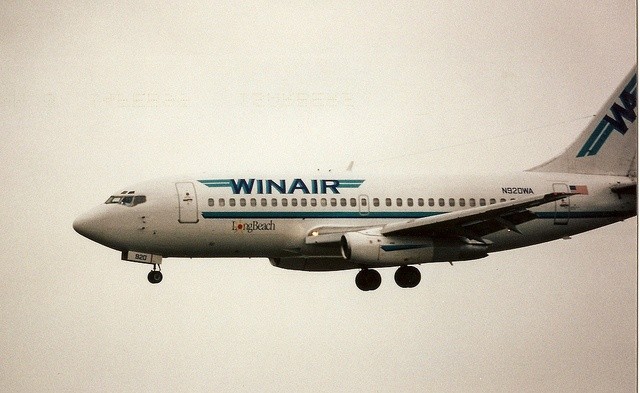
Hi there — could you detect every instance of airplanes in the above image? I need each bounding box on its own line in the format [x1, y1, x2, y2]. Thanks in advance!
[72, 62, 638, 291]
